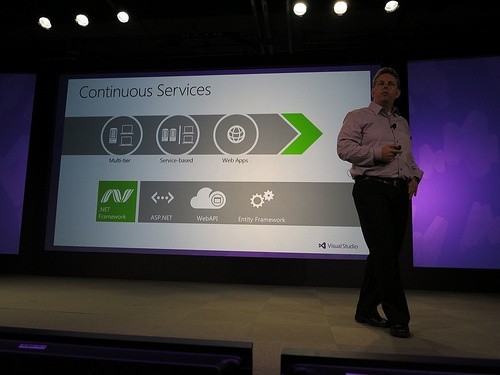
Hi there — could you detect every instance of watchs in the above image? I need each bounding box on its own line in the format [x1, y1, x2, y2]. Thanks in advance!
[412, 176, 420, 184]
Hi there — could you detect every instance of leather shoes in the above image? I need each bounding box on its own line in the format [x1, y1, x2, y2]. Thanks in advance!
[390, 320, 409, 338]
[356, 311, 391, 328]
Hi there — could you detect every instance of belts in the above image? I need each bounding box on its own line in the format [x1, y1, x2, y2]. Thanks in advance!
[356, 174, 407, 188]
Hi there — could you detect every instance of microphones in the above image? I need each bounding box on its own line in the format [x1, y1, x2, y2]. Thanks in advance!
[390, 123, 396, 128]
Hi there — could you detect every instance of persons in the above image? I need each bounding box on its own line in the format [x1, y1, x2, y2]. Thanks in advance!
[337, 68, 424, 339]
